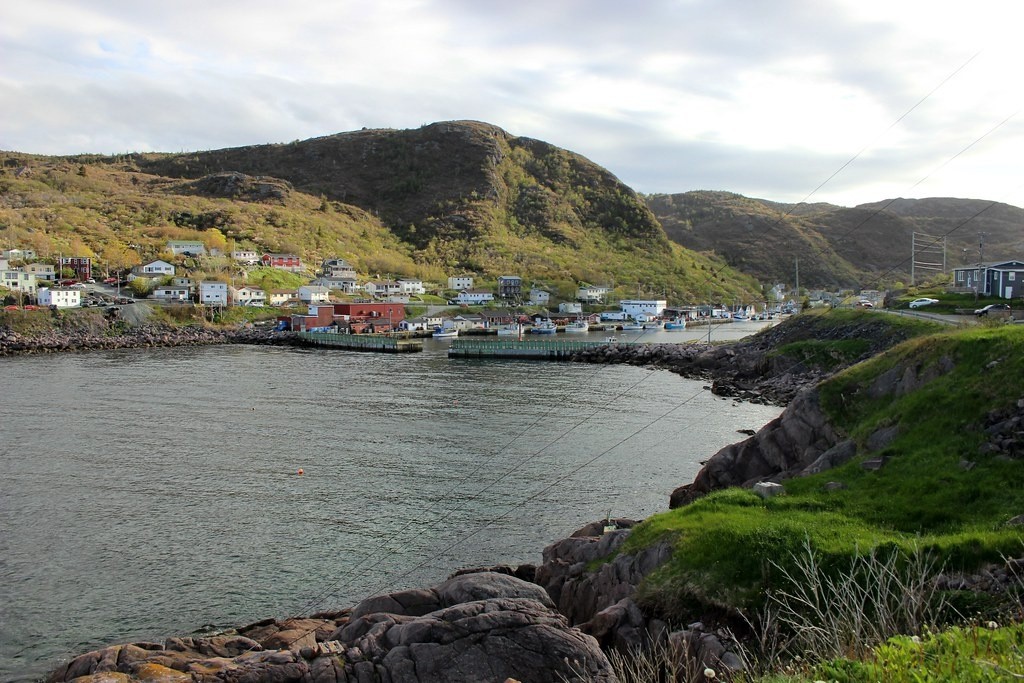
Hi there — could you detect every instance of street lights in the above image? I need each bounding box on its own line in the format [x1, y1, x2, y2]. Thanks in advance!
[389, 308, 393, 337]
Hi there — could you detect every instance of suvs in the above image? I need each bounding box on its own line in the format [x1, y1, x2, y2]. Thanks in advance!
[975, 304, 1010, 316]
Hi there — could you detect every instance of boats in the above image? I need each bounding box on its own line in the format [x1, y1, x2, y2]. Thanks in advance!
[643, 321, 664, 328]
[530, 320, 557, 334]
[432, 327, 459, 336]
[664, 315, 686, 329]
[565, 316, 589, 332]
[497, 322, 524, 336]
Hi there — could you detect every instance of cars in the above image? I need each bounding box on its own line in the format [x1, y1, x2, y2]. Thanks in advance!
[909, 298, 938, 308]
[857, 300, 873, 308]
